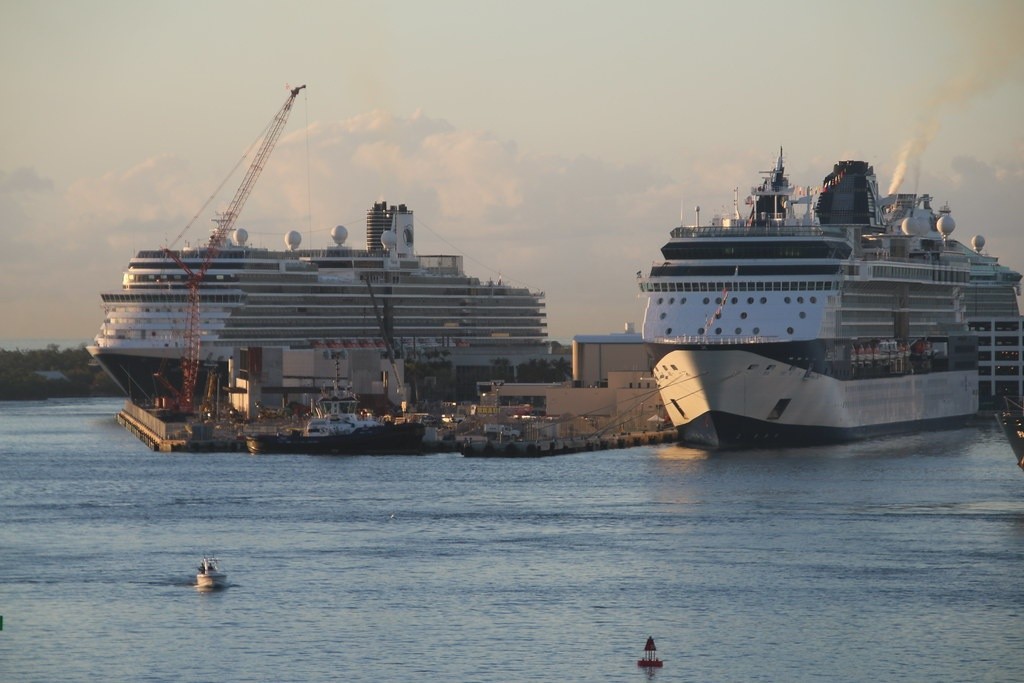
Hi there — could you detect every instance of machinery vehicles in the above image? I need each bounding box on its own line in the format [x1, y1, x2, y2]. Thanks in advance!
[197, 368, 218, 422]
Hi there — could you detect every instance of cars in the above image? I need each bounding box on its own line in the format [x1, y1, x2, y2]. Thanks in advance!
[407, 413, 439, 427]
[442, 414, 466, 424]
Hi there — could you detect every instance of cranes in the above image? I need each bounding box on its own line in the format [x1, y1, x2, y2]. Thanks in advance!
[151, 84, 309, 422]
[359, 274, 418, 424]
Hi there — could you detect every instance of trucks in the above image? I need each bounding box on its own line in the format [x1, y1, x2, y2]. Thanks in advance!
[483, 424, 520, 441]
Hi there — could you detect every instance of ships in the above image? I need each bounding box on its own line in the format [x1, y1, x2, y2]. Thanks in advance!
[202, 203, 574, 407]
[641, 146, 1024, 448]
[85, 225, 466, 400]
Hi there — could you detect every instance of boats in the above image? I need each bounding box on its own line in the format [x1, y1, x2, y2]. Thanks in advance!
[871, 342, 881, 368]
[990, 393, 1024, 473]
[244, 381, 427, 455]
[912, 337, 937, 361]
[849, 343, 857, 372]
[857, 342, 865, 372]
[878, 340, 912, 367]
[195, 553, 227, 592]
[864, 341, 873, 368]
